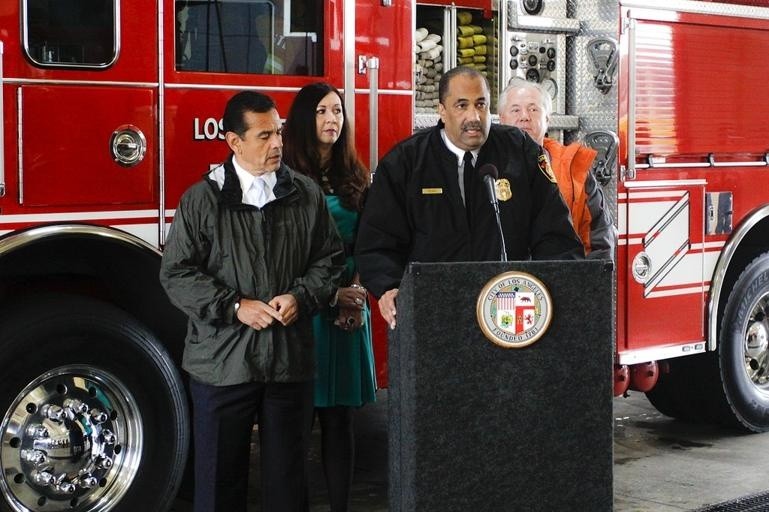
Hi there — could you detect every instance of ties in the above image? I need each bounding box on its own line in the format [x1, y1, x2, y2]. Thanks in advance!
[463, 152, 475, 222]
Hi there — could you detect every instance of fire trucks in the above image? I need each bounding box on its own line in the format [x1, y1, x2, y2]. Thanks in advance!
[1, 0, 768, 511]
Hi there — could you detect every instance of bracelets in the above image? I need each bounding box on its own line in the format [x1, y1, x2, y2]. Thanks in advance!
[350, 282, 365, 291]
[328, 288, 339, 309]
[234, 293, 244, 316]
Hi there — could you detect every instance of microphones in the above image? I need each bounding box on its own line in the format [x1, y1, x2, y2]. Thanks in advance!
[478, 161, 501, 218]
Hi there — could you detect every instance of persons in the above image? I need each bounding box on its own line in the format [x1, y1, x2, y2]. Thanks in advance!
[350, 66, 588, 332]
[158, 86, 349, 511]
[279, 82, 381, 512]
[498, 78, 616, 264]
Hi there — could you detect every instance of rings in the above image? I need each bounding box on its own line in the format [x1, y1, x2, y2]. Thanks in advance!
[346, 318, 356, 327]
[355, 297, 364, 305]
